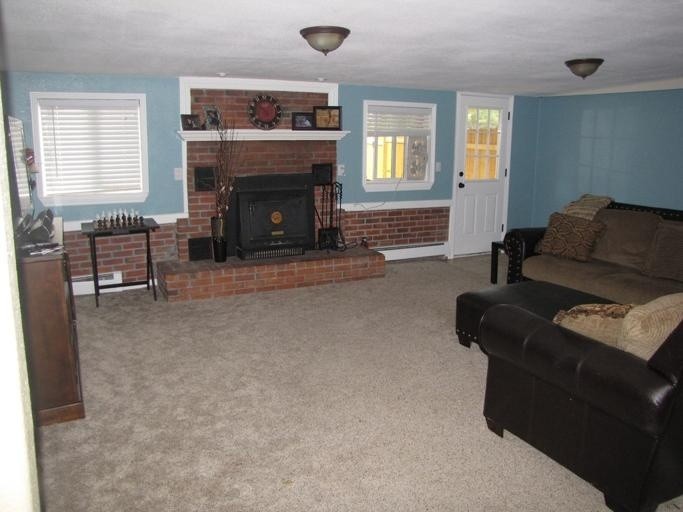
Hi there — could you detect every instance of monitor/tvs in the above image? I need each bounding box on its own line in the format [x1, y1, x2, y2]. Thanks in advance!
[4, 112, 33, 218]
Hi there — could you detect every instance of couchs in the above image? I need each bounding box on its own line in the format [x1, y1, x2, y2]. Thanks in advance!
[504, 194, 683, 306]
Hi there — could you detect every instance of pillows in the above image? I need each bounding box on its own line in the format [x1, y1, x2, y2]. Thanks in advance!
[534, 212, 605, 262]
[552, 304, 632, 348]
[621, 292, 683, 361]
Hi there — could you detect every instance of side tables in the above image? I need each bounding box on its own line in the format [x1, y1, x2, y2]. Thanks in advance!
[81, 218, 159, 307]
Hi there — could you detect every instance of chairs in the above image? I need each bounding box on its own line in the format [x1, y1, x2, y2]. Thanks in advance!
[477, 304, 683, 512]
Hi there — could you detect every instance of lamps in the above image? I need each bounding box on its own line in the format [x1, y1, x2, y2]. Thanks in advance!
[564, 58, 604, 80]
[300, 26, 351, 56]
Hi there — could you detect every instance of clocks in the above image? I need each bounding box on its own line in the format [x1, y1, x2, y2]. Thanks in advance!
[248, 95, 282, 129]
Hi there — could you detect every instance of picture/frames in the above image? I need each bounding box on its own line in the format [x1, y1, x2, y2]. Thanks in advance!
[292, 112, 314, 130]
[203, 105, 221, 130]
[313, 106, 342, 130]
[181, 114, 201, 130]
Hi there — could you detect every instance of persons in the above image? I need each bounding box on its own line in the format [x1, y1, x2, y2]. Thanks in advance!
[301, 116, 311, 127]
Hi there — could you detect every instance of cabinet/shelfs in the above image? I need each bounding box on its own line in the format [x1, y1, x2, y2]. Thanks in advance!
[22, 214, 85, 425]
